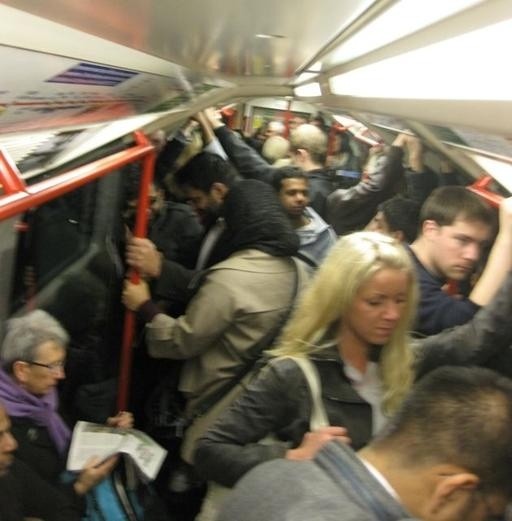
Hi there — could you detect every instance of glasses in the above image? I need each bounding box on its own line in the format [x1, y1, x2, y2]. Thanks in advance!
[30, 360, 65, 370]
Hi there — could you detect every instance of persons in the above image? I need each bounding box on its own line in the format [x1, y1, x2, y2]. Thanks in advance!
[2, 106, 512, 520]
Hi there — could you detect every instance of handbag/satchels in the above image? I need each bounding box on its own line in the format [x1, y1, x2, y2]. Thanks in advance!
[138, 386, 206, 493]
